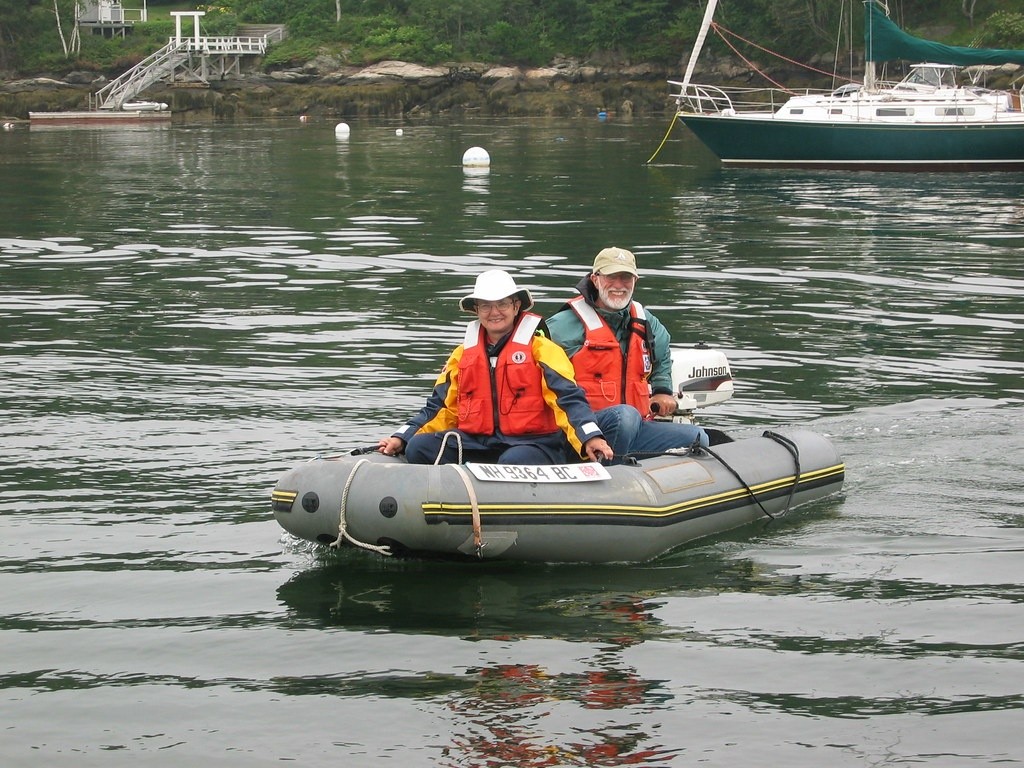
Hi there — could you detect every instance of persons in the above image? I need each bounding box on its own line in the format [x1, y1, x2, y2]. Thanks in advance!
[379, 269, 613, 465]
[545, 247, 709, 466]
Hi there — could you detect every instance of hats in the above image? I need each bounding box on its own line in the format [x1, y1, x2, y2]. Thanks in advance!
[459, 269, 534, 314]
[593, 246, 639, 279]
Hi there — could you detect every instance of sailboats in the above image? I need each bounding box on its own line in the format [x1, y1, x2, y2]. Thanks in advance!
[668, 0, 1024, 174]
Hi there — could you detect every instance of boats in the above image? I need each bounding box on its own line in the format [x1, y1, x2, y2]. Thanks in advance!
[122, 100, 169, 111]
[275, 340, 845, 568]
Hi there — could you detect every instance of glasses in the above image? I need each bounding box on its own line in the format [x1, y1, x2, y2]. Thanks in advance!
[596, 274, 633, 282]
[474, 300, 518, 313]
